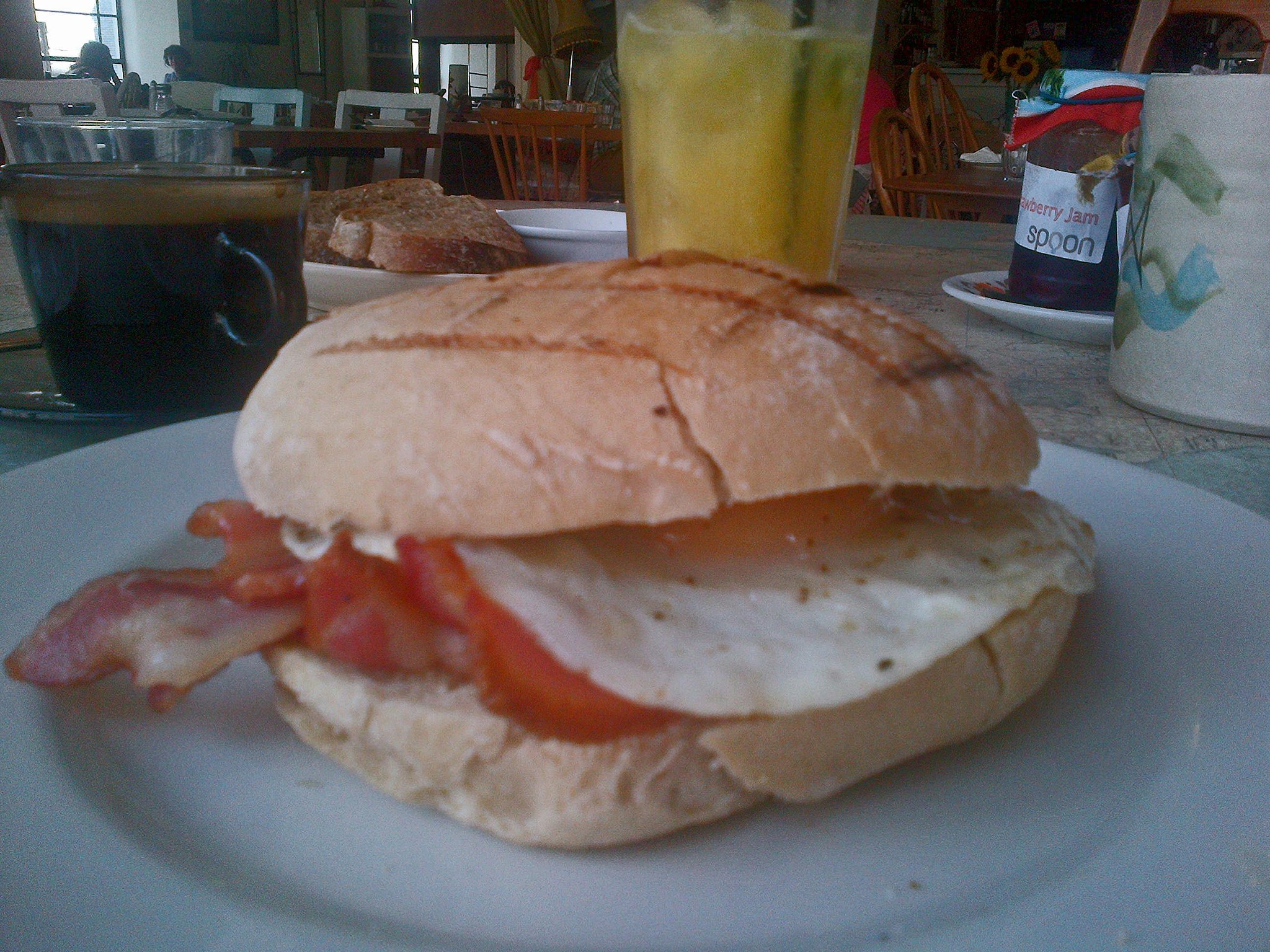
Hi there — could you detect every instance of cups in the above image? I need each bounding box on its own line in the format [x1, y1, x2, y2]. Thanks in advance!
[615, 1, 874, 289]
[3, 97, 317, 409]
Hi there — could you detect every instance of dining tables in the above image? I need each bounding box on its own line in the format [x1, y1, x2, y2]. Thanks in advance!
[1, 199, 1268, 952]
[884, 161, 1020, 223]
[443, 116, 621, 144]
[227, 122, 442, 190]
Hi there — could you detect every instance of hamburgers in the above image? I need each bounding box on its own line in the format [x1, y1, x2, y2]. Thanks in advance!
[6, 249, 1092, 851]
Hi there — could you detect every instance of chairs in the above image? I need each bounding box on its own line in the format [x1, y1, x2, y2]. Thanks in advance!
[866, 104, 952, 221]
[323, 88, 449, 192]
[0, 75, 132, 167]
[908, 56, 985, 221]
[213, 86, 313, 179]
[478, 102, 597, 205]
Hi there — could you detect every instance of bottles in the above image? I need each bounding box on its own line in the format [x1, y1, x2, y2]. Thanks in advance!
[1016, 39, 1270, 433]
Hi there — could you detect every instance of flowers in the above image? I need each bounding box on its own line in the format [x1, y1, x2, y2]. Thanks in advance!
[977, 36, 1062, 92]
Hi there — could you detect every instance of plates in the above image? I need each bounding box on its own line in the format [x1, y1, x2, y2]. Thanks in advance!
[296, 207, 633, 330]
[0, 390, 1268, 948]
[938, 256, 1119, 347]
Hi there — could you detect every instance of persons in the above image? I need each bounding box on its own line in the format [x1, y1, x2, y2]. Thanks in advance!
[68, 40, 119, 90]
[850, 68, 898, 209]
[495, 80, 516, 107]
[163, 44, 204, 82]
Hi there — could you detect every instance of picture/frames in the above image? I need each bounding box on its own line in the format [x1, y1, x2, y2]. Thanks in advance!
[191, 0, 280, 48]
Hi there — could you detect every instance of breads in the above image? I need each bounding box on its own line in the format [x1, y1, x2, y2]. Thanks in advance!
[307, 176, 538, 273]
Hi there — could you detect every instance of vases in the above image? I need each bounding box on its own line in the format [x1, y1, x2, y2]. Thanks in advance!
[1005, 87, 1028, 135]
